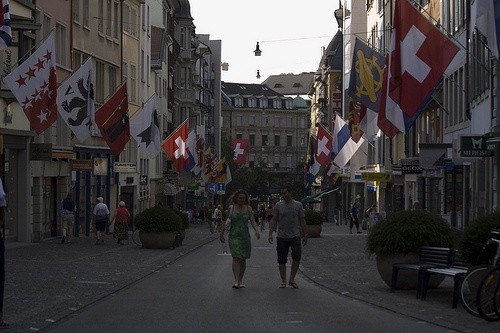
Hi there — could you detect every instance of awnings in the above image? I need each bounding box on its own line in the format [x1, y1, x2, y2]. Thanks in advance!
[164, 183, 178, 195]
[300, 196, 320, 204]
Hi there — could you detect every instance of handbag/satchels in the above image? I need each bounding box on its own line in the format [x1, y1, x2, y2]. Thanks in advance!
[217, 217, 222, 222]
[225, 205, 234, 230]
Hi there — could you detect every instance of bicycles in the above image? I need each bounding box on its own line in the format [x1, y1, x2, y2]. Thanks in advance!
[494, 282, 500, 317]
[459, 228, 500, 319]
[475, 238, 500, 322]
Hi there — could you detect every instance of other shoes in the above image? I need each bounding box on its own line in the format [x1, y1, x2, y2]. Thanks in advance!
[357, 231, 362, 234]
[239, 284, 246, 288]
[280, 284, 286, 288]
[102, 239, 104, 243]
[117, 239, 121, 244]
[350, 232, 352, 234]
[97, 238, 100, 245]
[61, 236, 66, 244]
[120, 243, 124, 245]
[68, 241, 73, 245]
[232, 284, 238, 289]
[289, 282, 299, 288]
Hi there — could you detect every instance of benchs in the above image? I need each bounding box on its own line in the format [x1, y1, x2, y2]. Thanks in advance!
[390, 246, 451, 298]
[420, 248, 472, 309]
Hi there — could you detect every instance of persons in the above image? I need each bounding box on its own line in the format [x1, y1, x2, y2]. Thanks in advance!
[304, 202, 310, 214]
[191, 203, 227, 234]
[268, 186, 308, 288]
[61, 192, 77, 245]
[333, 207, 340, 225]
[363, 202, 376, 230]
[413, 201, 420, 212]
[253, 203, 273, 232]
[92, 197, 110, 245]
[220, 189, 260, 289]
[110, 200, 131, 245]
[350, 202, 364, 234]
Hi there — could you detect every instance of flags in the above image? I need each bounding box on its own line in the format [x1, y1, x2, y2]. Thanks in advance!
[0, 0, 12, 50]
[3, 28, 58, 135]
[56, 56, 95, 143]
[96, 81, 130, 157]
[129, 93, 161, 159]
[474, 0, 500, 60]
[162, 119, 190, 172]
[196, 137, 232, 186]
[232, 139, 248, 164]
[302, 111, 366, 188]
[348, 0, 461, 141]
[184, 131, 198, 173]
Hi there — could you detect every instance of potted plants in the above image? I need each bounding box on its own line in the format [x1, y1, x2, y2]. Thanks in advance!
[135, 207, 189, 250]
[366, 209, 450, 289]
[304, 211, 324, 237]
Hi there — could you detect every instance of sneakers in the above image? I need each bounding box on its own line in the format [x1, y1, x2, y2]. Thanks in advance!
[0, 319, 10, 328]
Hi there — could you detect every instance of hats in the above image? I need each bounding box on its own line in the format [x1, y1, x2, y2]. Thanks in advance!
[68, 192, 72, 197]
